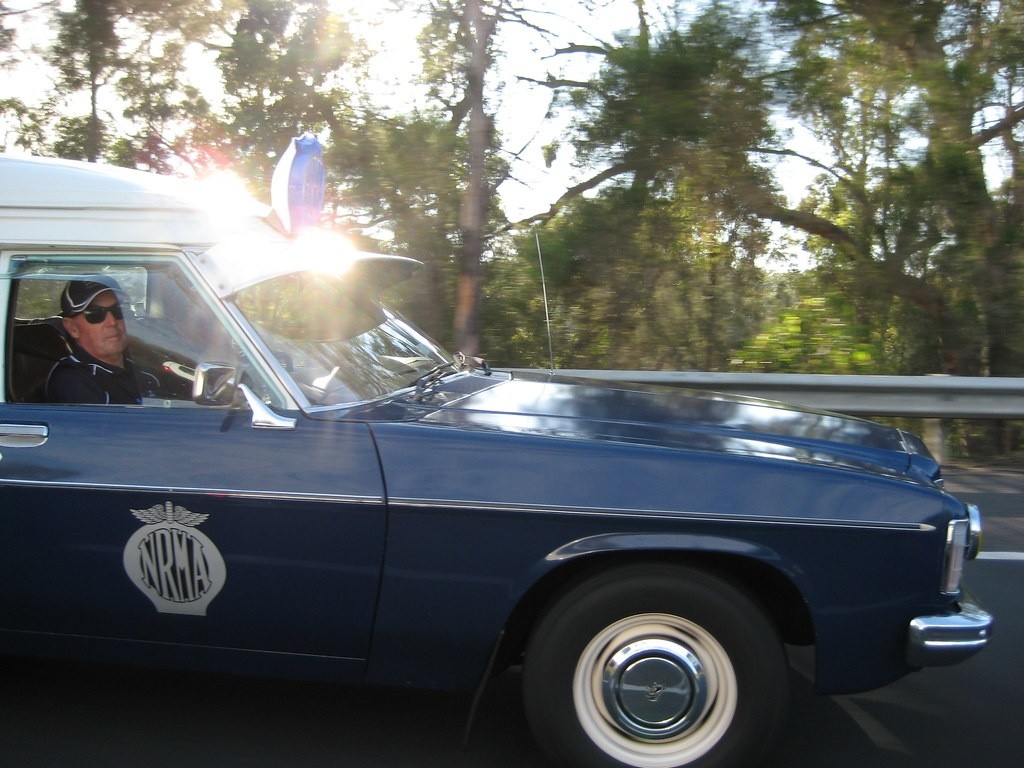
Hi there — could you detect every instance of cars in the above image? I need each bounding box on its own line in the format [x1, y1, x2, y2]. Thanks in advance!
[0, 150, 998, 768]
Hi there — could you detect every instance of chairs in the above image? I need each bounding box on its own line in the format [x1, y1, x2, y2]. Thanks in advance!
[10, 323, 74, 406]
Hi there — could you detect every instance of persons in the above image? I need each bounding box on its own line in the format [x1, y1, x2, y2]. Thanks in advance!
[41, 272, 186, 404]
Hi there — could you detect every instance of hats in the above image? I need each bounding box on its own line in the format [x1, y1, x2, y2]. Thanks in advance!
[60, 273, 129, 317]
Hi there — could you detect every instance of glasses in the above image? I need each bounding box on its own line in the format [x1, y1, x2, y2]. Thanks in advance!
[69, 303, 131, 324]
